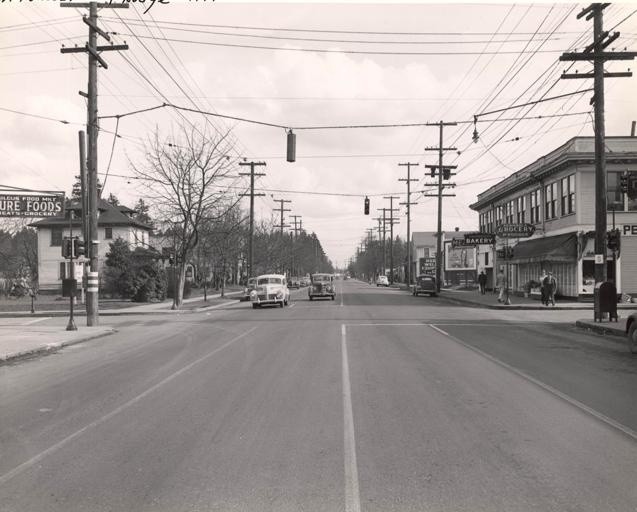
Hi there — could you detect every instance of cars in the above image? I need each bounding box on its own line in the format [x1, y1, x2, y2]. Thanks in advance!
[624, 311, 637, 354]
[376, 275, 389, 287]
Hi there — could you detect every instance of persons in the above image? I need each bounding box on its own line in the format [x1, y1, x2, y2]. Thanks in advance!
[491, 268, 507, 304]
[476, 270, 487, 295]
[539, 268, 547, 303]
[541, 271, 557, 307]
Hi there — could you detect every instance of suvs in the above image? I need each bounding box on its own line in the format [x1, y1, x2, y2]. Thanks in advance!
[239, 272, 351, 310]
[411, 275, 435, 297]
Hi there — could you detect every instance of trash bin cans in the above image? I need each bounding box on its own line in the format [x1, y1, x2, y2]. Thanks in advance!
[594, 282, 618, 322]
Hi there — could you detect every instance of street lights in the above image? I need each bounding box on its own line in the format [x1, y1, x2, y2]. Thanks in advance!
[609, 204, 615, 283]
[468, 87, 609, 316]
[64, 209, 77, 331]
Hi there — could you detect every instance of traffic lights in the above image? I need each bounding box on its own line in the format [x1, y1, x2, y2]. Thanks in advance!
[606, 227, 620, 250]
[60, 239, 71, 257]
[619, 171, 628, 193]
[176, 250, 183, 263]
[168, 252, 174, 264]
[364, 199, 369, 215]
[506, 246, 514, 258]
[73, 239, 85, 257]
